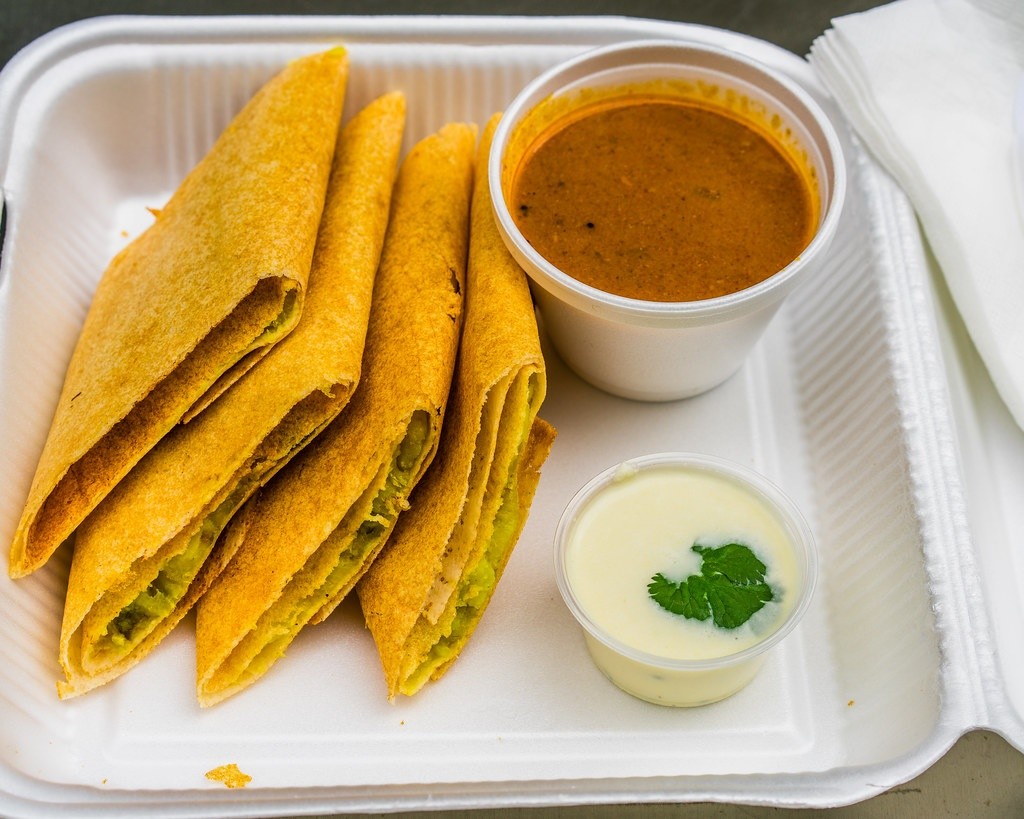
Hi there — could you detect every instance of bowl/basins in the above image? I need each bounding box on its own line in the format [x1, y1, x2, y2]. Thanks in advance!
[489, 41, 846, 403]
[552, 453, 817, 708]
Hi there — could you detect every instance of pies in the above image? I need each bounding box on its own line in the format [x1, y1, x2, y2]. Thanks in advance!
[9, 44, 559, 706]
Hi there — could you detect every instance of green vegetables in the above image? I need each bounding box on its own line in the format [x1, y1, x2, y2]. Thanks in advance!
[647, 540, 772, 627]
